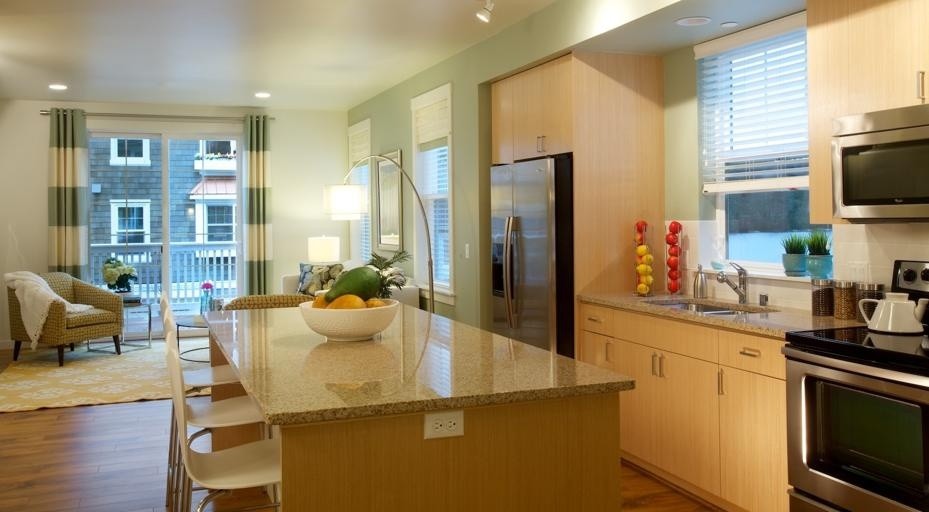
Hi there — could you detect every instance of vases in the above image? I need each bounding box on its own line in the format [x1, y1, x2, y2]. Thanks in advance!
[199, 290, 214, 316]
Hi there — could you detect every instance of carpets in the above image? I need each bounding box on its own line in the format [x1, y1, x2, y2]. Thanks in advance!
[0, 338, 212, 412]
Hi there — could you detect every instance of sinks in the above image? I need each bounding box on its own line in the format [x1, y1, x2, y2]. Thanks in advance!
[648, 302, 732, 313]
[705, 310, 763, 317]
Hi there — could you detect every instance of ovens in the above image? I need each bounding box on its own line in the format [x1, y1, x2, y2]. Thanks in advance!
[785, 359, 927, 512]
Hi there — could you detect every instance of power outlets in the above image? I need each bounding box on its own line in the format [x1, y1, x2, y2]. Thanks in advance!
[423, 410, 464, 439]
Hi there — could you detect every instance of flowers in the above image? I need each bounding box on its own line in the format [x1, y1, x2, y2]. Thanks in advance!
[199, 280, 214, 290]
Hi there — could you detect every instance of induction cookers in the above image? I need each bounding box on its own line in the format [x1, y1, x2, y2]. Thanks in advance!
[784, 325, 929, 376]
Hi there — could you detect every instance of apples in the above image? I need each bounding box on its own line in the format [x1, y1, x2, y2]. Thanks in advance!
[666, 221, 682, 294]
[635, 222, 653, 294]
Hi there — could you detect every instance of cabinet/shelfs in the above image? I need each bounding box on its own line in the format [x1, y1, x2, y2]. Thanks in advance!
[580, 300, 612, 366]
[612, 303, 720, 512]
[721, 328, 789, 512]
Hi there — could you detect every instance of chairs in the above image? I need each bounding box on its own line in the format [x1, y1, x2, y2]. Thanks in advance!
[166, 362, 284, 512]
[159, 296, 238, 391]
[6, 269, 126, 364]
[163, 330, 271, 510]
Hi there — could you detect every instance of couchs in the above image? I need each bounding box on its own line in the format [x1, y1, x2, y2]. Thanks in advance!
[281, 259, 419, 308]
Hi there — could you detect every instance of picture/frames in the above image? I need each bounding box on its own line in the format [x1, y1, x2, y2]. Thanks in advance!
[374, 150, 408, 254]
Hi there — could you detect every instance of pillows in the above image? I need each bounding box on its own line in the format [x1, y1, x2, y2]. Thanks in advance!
[293, 261, 341, 297]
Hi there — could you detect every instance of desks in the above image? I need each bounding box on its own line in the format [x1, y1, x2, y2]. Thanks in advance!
[215, 301, 634, 512]
[90, 294, 154, 356]
[174, 317, 211, 362]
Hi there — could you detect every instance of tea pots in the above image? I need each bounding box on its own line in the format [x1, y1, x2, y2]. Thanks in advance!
[858, 292, 928, 335]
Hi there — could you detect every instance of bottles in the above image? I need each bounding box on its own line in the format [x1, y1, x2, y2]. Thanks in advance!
[199, 283, 213, 313]
[694, 272, 707, 298]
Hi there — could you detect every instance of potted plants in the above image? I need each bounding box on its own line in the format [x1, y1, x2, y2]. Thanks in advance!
[803, 228, 834, 278]
[103, 260, 137, 294]
[779, 232, 811, 275]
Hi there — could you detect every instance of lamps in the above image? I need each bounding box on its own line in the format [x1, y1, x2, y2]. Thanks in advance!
[335, 155, 441, 315]
[305, 233, 338, 263]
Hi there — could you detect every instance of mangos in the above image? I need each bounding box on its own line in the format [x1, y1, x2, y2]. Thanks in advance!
[326, 267, 381, 301]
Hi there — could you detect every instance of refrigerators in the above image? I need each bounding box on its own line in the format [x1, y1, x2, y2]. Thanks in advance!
[488, 156, 559, 356]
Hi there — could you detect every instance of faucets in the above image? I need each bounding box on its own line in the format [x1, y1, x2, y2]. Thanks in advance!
[717, 261, 747, 304]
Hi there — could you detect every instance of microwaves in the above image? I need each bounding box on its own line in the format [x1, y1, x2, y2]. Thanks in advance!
[831, 105, 929, 218]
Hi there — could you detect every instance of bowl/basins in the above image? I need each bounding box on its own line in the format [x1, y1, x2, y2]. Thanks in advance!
[300, 297, 400, 342]
